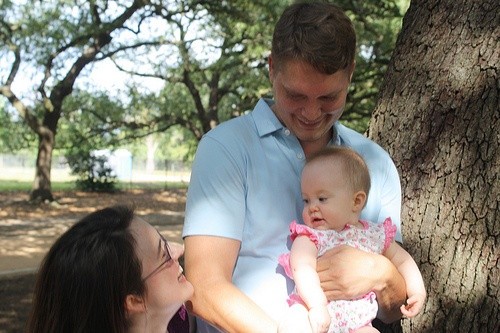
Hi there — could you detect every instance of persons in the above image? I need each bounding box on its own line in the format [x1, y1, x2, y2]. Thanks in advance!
[181, 1, 403, 332]
[28, 204, 197, 333]
[276, 148, 427, 333]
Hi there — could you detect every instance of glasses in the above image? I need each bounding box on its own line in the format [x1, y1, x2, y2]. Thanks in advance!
[133, 228, 176, 287]
